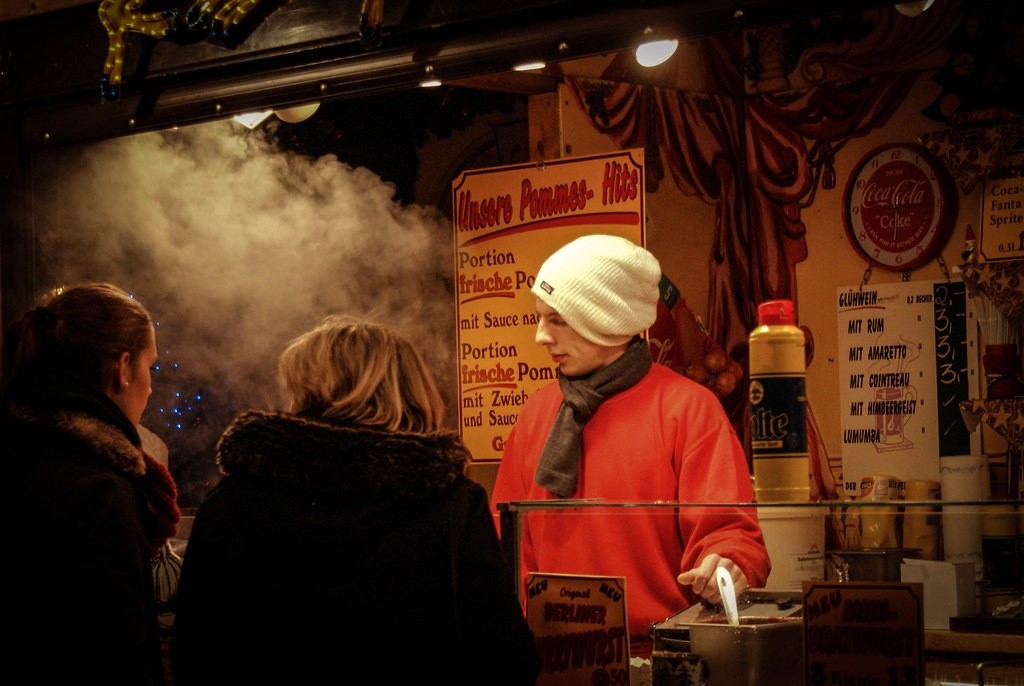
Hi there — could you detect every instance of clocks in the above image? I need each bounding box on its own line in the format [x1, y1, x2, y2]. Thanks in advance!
[842, 143, 946, 270]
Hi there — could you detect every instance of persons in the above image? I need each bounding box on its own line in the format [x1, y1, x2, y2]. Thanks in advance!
[172, 317, 539, 685]
[0, 282, 180, 685]
[493, 234, 773, 662]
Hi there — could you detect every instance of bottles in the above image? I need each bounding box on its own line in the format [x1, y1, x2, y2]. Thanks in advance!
[750, 300, 810, 504]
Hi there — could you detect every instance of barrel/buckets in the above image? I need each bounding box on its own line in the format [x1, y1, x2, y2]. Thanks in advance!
[757, 507, 830, 591]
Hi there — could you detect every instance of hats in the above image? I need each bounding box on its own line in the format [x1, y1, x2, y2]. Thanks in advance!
[529, 233, 663, 347]
